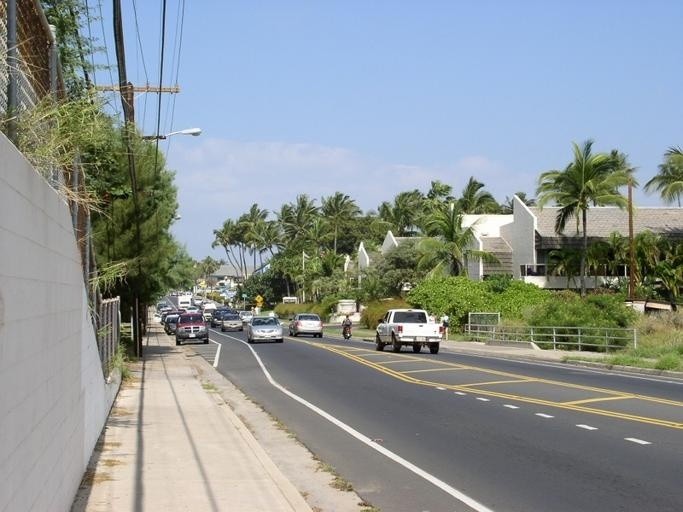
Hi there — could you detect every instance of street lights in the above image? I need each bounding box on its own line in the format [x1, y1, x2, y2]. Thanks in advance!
[150, 128, 202, 142]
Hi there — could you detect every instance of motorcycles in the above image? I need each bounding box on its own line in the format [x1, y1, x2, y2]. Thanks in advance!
[343, 327, 352, 340]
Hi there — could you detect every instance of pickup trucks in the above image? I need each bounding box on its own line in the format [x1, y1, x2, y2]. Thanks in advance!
[376, 308, 444, 353]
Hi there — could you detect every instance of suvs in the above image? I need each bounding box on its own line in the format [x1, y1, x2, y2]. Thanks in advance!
[176, 313, 208, 345]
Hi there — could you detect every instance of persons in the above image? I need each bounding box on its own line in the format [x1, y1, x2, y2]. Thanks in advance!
[429, 313, 437, 324]
[342, 315, 352, 334]
[440, 312, 450, 341]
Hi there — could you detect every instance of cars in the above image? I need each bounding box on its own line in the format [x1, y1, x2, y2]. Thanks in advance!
[158, 292, 253, 335]
[248, 316, 283, 343]
[289, 314, 323, 338]
